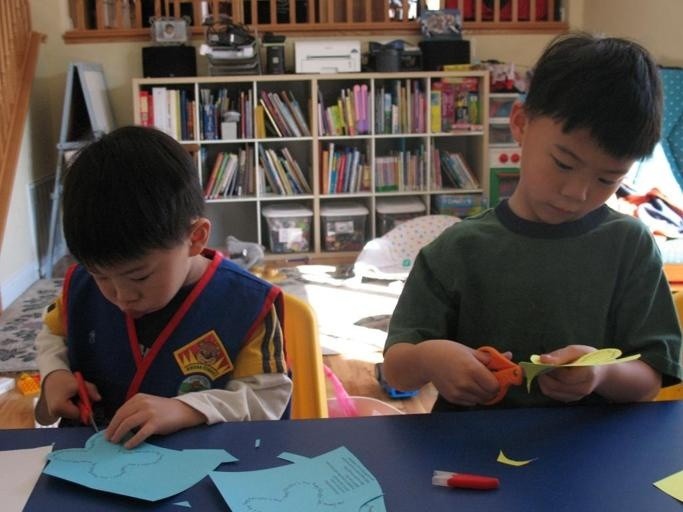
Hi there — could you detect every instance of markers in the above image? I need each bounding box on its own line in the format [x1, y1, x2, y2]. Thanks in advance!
[431, 469, 500, 491]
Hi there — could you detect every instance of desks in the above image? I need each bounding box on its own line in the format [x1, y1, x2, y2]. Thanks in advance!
[0, 400, 683, 512]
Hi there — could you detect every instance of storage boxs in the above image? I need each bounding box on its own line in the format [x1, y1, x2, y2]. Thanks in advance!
[487, 93, 520, 148]
[261, 198, 425, 255]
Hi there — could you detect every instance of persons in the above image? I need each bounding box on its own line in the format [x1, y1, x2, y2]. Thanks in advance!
[423, 14, 459, 36]
[159, 21, 181, 39]
[381, 34, 679, 415]
[32, 124, 291, 449]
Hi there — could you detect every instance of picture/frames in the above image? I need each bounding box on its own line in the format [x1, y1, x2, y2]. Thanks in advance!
[147, 14, 190, 51]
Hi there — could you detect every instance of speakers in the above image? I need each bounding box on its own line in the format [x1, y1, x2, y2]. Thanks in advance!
[141, 45, 197, 77]
[418, 39, 471, 72]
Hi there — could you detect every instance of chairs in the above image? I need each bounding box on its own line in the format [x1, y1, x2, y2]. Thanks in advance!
[278, 292, 328, 424]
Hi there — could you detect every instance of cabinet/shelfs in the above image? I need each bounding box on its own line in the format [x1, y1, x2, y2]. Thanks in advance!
[129, 70, 495, 260]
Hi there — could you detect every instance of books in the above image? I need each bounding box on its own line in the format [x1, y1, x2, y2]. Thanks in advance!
[189, 142, 480, 200]
[375, 76, 483, 133]
[259, 84, 371, 138]
[139, 87, 253, 139]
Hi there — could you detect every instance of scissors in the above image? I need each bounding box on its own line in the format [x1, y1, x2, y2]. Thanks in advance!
[476, 346, 550, 406]
[73, 371, 101, 436]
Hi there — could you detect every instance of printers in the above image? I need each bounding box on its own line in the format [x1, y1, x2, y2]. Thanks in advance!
[294, 39, 361, 73]
